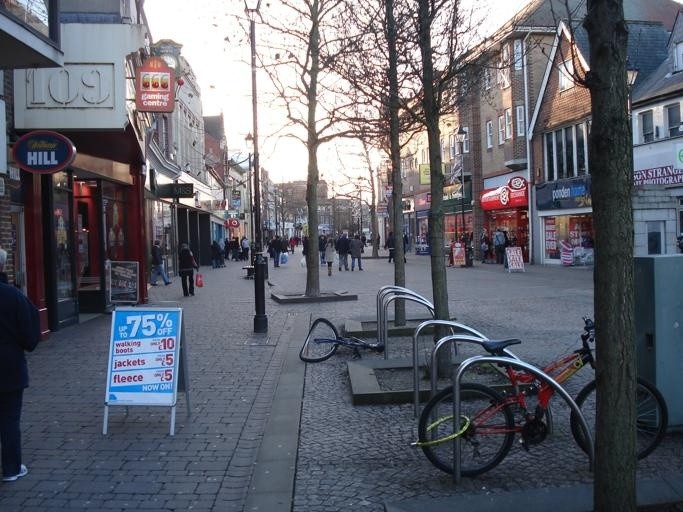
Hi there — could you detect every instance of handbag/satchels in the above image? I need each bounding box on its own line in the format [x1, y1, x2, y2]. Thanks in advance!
[301, 256, 306, 267]
[196, 274, 203, 286]
[481, 244, 488, 251]
[496, 246, 504, 252]
[281, 252, 287, 264]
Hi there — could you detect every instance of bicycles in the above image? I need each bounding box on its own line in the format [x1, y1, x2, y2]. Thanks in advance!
[299, 317, 385, 363]
[418, 315, 668, 478]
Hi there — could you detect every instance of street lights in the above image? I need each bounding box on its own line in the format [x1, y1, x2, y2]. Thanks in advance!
[245, 133, 254, 267]
[244, 0, 269, 331]
[455, 127, 466, 240]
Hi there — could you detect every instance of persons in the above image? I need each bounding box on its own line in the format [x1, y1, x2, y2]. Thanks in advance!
[493, 228, 505, 264]
[178, 241, 199, 297]
[208, 231, 410, 272]
[0, 248, 42, 482]
[151, 239, 173, 286]
[500, 231, 510, 262]
[479, 227, 491, 263]
[323, 238, 337, 277]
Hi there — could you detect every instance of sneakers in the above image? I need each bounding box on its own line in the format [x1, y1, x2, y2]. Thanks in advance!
[184, 290, 194, 296]
[339, 267, 363, 271]
[150, 282, 172, 285]
[275, 265, 280, 267]
[3, 464, 27, 481]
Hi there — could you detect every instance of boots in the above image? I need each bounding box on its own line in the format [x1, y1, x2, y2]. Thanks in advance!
[328, 267, 331, 276]
